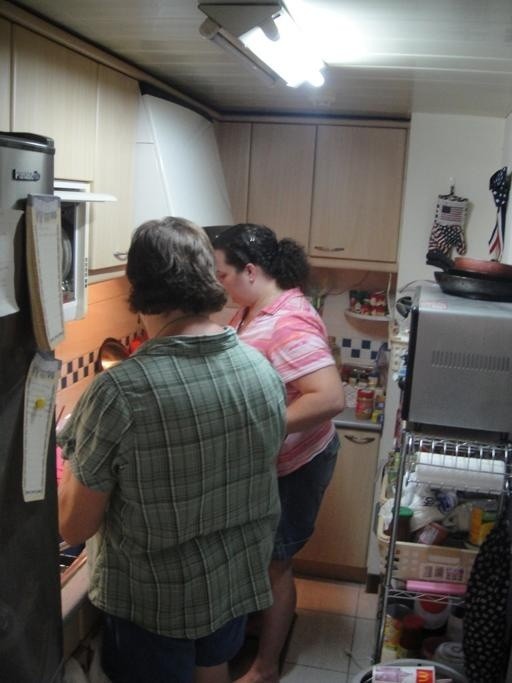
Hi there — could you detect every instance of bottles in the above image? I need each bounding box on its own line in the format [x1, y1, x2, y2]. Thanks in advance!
[347, 366, 383, 424]
[383, 447, 402, 498]
[397, 611, 422, 660]
[386, 506, 414, 542]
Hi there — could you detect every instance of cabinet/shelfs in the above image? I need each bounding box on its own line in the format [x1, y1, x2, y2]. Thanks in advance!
[0, 2, 100, 182]
[285, 399, 382, 583]
[218, 108, 411, 275]
[366, 387, 509, 668]
[82, 44, 145, 287]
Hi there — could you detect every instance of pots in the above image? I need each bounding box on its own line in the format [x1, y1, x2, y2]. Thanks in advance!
[431, 272, 512, 303]
[96, 336, 132, 373]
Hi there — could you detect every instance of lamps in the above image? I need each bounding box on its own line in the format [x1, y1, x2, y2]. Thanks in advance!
[190, 0, 332, 92]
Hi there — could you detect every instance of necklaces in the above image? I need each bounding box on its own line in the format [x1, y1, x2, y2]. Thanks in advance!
[155, 311, 206, 338]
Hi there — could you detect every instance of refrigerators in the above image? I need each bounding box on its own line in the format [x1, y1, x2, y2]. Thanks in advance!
[1, 128, 65, 680]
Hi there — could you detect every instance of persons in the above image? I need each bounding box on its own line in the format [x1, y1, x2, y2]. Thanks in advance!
[209, 222, 348, 683]
[59, 213, 290, 683]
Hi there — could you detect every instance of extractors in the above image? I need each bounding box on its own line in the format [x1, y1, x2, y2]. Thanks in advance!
[136, 81, 237, 252]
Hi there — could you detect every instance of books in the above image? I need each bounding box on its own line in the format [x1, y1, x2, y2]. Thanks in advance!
[25, 192, 68, 351]
